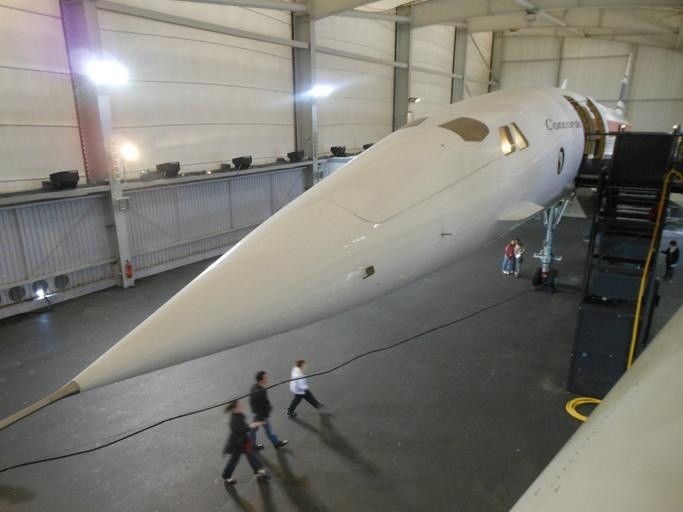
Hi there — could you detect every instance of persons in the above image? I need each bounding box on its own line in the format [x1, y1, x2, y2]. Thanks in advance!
[502, 239, 515, 274]
[222, 400, 268, 484]
[510, 238, 526, 278]
[286, 360, 323, 418]
[249, 371, 288, 449]
[660, 241, 679, 283]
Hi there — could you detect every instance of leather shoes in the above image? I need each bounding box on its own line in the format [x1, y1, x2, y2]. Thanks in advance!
[252, 443, 264, 450]
[274, 440, 288, 449]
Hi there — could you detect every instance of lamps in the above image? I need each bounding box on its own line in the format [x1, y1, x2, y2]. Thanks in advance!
[408, 98, 421, 102]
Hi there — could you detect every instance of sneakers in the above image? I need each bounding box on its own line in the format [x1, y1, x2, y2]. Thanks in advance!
[286, 410, 298, 416]
[256, 475, 272, 480]
[224, 479, 236, 484]
[316, 403, 324, 409]
